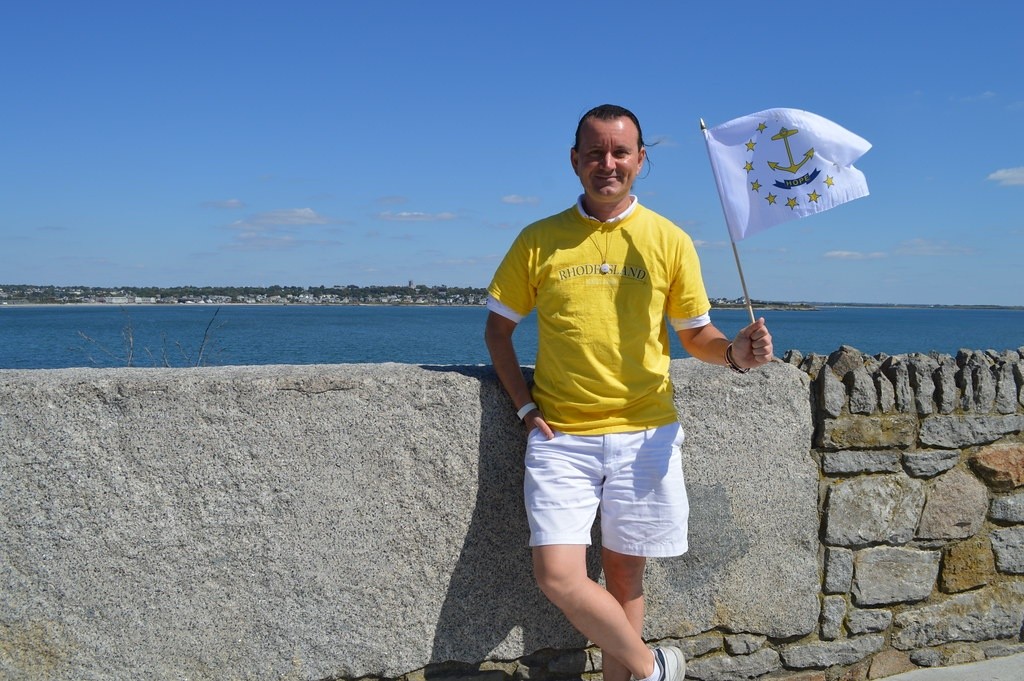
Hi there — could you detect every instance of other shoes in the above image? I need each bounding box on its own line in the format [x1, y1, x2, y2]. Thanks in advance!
[651, 645, 686, 681]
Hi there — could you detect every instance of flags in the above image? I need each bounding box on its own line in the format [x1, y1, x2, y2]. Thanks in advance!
[707, 107, 874, 244]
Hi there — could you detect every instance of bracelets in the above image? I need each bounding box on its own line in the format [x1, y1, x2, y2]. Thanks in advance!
[725, 342, 751, 374]
[517, 402, 538, 420]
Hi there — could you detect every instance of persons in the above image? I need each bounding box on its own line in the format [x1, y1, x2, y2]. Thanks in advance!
[485, 102, 775, 681]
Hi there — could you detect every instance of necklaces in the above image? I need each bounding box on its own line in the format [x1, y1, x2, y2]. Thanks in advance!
[589, 220, 614, 274]
